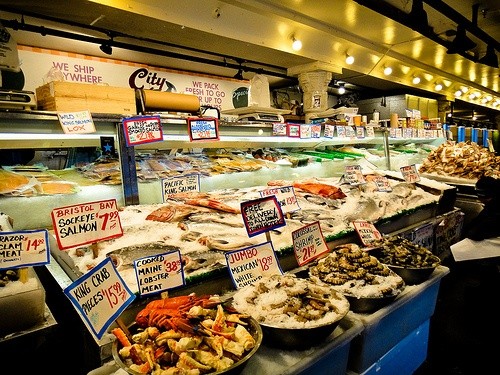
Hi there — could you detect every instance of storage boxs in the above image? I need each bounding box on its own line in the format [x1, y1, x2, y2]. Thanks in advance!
[36, 80, 137, 115]
[86, 265, 450, 375]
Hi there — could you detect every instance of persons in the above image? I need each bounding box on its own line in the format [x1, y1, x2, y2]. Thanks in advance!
[460, 176, 500, 241]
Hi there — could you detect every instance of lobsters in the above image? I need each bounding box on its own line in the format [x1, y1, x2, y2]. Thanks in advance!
[136, 294, 213, 333]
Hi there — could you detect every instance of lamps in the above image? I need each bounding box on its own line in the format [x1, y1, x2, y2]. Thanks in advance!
[447, 23, 499, 69]
[233, 58, 248, 81]
[99, 30, 119, 55]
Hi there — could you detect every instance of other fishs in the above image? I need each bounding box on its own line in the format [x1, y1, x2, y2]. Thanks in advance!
[75, 139, 500, 272]
